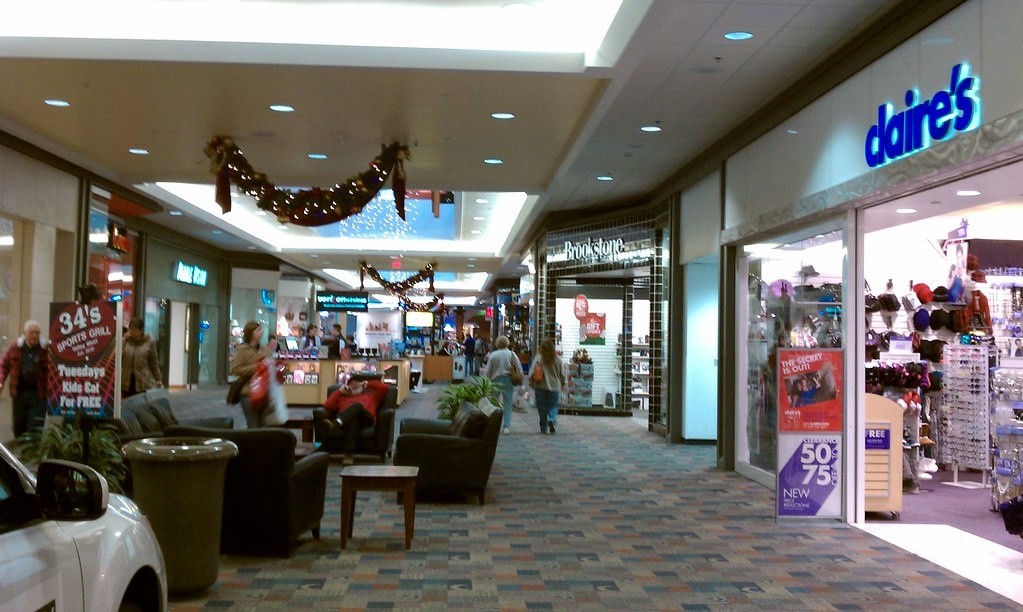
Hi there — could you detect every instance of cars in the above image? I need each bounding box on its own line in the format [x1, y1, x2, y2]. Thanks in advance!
[0, 443, 168, 612]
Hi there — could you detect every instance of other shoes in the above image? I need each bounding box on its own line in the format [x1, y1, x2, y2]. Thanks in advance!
[864, 359, 943, 481]
[541, 428, 547, 433]
[503, 427, 510, 434]
[342, 453, 353, 467]
[322, 419, 338, 437]
[548, 421, 557, 433]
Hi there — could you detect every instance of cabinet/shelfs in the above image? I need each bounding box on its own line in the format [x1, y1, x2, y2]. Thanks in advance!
[990, 355, 1023, 513]
[614, 342, 649, 396]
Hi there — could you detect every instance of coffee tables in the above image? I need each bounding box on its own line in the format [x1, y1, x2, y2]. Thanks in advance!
[293, 441, 322, 457]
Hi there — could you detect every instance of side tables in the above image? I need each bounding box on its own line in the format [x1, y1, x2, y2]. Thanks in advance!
[338, 464, 421, 554]
[273, 418, 316, 443]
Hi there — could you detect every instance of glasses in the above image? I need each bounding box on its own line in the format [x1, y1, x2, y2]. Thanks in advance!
[934, 330, 1002, 471]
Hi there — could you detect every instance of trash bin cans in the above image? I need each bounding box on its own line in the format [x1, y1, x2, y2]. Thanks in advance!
[121, 436, 238, 601]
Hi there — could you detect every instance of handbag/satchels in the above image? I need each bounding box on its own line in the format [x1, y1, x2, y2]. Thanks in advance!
[226, 355, 290, 427]
[532, 356, 544, 382]
[511, 351, 523, 387]
[748, 261, 923, 355]
[1000, 497, 1023, 536]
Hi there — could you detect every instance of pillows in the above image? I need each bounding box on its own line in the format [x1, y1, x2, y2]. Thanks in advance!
[447, 402, 486, 438]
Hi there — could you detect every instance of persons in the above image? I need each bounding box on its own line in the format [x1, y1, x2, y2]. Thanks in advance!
[121, 317, 163, 400]
[402, 353, 421, 393]
[463, 332, 475, 377]
[230, 321, 276, 429]
[0, 320, 48, 455]
[473, 333, 483, 376]
[299, 323, 357, 359]
[268, 334, 281, 353]
[791, 376, 821, 408]
[438, 341, 453, 357]
[312, 374, 391, 453]
[484, 335, 523, 435]
[528, 337, 567, 434]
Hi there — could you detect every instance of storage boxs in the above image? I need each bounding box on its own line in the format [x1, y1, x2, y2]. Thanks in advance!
[564, 363, 594, 407]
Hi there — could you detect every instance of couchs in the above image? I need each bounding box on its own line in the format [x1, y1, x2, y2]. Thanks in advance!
[312, 384, 400, 464]
[394, 396, 504, 508]
[162, 424, 329, 552]
[120, 398, 234, 439]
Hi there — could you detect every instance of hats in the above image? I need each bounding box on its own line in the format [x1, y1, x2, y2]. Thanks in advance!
[971, 271, 987, 284]
[967, 249, 979, 273]
[911, 282, 972, 396]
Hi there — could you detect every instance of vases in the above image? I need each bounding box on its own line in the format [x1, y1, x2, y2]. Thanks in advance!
[122, 438, 241, 601]
